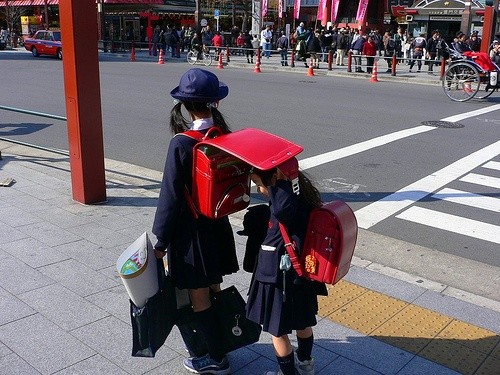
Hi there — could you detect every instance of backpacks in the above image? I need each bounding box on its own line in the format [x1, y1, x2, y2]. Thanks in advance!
[168, 125, 304, 217]
[279, 194, 358, 285]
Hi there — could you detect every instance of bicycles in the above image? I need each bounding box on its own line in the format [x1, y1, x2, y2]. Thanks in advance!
[187, 48, 212, 66]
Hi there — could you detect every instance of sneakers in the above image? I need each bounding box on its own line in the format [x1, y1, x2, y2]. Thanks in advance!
[265, 368, 284, 375]
[183, 353, 230, 375]
[294, 352, 314, 375]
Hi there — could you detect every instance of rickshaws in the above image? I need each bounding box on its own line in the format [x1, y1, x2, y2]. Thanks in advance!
[439, 40, 500, 101]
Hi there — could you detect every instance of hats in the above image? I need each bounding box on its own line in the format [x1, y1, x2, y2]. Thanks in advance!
[170, 67, 229, 99]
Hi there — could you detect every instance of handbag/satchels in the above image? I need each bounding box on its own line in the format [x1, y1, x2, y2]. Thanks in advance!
[129, 256, 175, 358]
[174, 285, 262, 357]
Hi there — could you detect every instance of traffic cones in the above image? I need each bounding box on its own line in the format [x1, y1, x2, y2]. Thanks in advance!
[369, 61, 379, 83]
[253, 53, 261, 73]
[216, 54, 224, 69]
[305, 59, 316, 76]
[157, 49, 165, 65]
[130, 47, 136, 62]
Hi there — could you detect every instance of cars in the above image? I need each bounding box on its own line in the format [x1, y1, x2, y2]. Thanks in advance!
[24, 30, 62, 61]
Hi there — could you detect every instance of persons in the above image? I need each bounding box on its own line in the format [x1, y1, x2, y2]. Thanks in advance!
[242, 166, 328, 375]
[151, 67, 240, 375]
[152, 20, 500, 92]
[1, 26, 22, 48]
